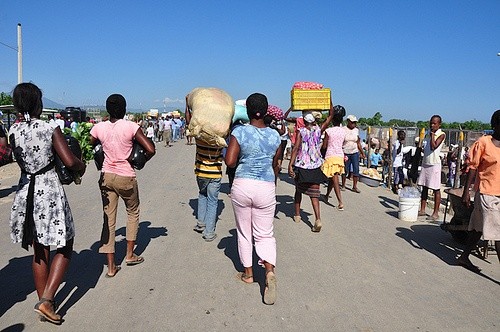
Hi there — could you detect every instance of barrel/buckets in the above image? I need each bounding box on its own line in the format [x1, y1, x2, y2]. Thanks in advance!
[397, 197, 421, 222]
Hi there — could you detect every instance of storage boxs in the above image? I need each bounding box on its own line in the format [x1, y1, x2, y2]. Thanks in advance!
[146, 110, 180, 120]
[291, 88, 331, 112]
[397, 188, 421, 222]
[359, 171, 383, 187]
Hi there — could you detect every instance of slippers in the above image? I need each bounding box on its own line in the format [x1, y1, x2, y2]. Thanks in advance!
[321, 194, 344, 211]
[105, 255, 144, 278]
[418, 211, 439, 220]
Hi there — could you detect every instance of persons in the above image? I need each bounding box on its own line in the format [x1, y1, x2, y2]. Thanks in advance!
[458, 110, 500, 273]
[417, 114, 446, 221]
[185, 96, 233, 242]
[267, 105, 421, 233]
[89, 93, 156, 278]
[138, 109, 194, 146]
[226, 92, 282, 306]
[8, 82, 87, 325]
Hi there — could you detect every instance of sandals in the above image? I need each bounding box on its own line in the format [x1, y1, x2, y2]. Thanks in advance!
[33, 297, 63, 324]
[263, 271, 278, 305]
[235, 272, 254, 287]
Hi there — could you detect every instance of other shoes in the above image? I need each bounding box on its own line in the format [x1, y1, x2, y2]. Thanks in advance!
[193, 225, 218, 241]
[293, 215, 322, 232]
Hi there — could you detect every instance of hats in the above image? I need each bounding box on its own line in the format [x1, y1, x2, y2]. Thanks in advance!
[347, 115, 358, 122]
